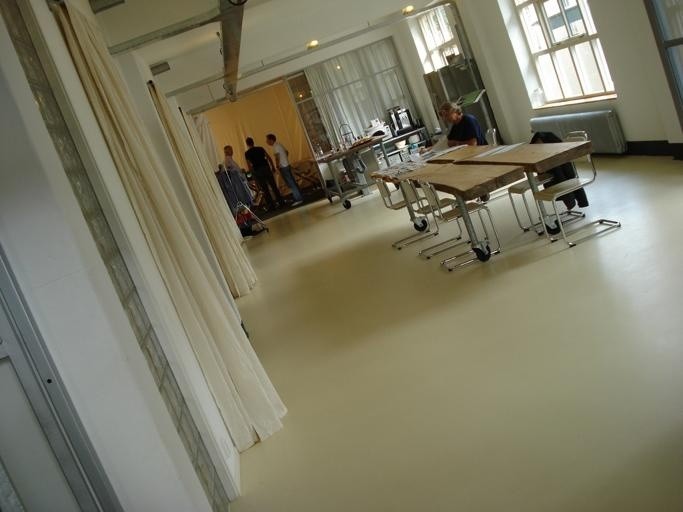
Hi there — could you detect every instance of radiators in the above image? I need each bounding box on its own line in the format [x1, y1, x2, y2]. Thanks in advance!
[530, 109, 629, 154]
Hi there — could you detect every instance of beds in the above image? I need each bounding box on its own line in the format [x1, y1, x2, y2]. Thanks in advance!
[256, 172, 322, 212]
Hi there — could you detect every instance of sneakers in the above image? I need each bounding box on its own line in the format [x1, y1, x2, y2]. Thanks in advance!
[291, 201, 304, 207]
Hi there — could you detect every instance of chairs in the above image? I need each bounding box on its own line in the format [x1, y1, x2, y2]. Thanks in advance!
[376, 128, 621, 272]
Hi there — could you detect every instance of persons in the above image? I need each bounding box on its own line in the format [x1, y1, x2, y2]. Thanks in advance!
[245, 137, 286, 211]
[224, 146, 255, 202]
[266, 134, 302, 207]
[418, 100, 490, 201]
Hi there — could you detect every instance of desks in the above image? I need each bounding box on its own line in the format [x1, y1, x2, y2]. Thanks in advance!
[308, 136, 399, 210]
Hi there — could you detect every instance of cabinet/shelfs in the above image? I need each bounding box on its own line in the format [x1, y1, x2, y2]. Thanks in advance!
[341, 126, 432, 196]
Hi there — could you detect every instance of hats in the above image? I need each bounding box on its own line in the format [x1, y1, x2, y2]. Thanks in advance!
[438, 102, 462, 118]
